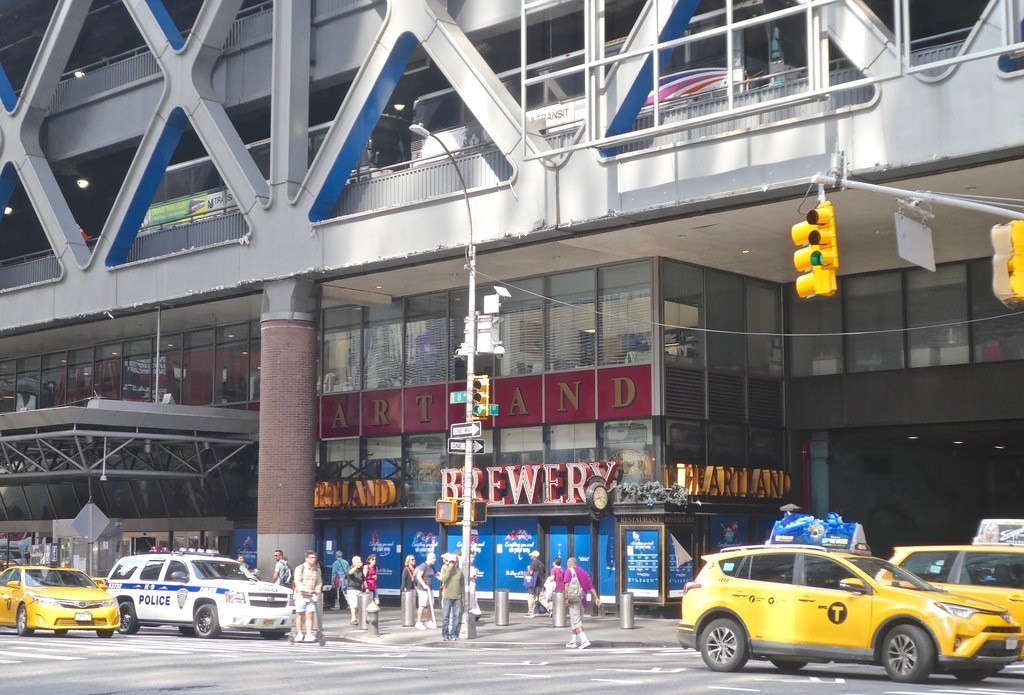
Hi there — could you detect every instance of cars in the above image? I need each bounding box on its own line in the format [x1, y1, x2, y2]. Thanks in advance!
[675, 543, 1024, 685]
[875, 518, 1024, 667]
[106, 548, 295, 639]
[0, 565, 121, 640]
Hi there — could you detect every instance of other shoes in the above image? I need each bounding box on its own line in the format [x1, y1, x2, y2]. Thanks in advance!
[524, 614, 534, 618]
[549, 610, 553, 617]
[443, 634, 449, 641]
[566, 642, 577, 648]
[451, 634, 457, 641]
[305, 634, 315, 642]
[427, 620, 437, 629]
[415, 622, 426, 630]
[351, 619, 358, 625]
[295, 634, 304, 641]
[579, 641, 591, 649]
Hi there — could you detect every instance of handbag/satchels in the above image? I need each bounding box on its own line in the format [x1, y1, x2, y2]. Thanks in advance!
[567, 567, 584, 602]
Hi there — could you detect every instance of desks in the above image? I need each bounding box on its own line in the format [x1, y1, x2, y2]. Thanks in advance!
[812, 354, 843, 375]
[901, 345, 970, 368]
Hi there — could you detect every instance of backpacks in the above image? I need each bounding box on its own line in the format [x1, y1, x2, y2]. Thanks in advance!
[412, 563, 426, 582]
[278, 562, 293, 588]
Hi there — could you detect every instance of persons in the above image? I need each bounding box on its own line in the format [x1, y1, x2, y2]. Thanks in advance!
[329, 551, 377, 626]
[523, 550, 565, 618]
[236, 554, 251, 573]
[271, 550, 289, 587]
[563, 557, 602, 649]
[293, 549, 322, 643]
[401, 550, 482, 642]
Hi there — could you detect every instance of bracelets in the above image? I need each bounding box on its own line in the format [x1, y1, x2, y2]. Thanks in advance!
[565, 597, 568, 600]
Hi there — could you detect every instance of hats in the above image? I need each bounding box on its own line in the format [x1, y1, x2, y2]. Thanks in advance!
[529, 550, 540, 557]
[441, 553, 450, 560]
[450, 554, 457, 561]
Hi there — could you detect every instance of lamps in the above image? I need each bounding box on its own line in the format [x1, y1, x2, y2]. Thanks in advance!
[223, 367, 228, 384]
[76, 177, 90, 188]
[144, 439, 151, 455]
[4, 206, 13, 215]
[84, 435, 94, 444]
[75, 69, 85, 78]
[201, 441, 212, 452]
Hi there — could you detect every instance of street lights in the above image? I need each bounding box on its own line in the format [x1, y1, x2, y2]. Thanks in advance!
[408, 123, 475, 638]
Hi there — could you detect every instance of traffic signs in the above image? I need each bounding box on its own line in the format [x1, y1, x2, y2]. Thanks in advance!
[451, 421, 481, 438]
[447, 438, 485, 455]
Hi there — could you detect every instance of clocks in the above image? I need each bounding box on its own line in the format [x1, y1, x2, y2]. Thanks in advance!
[587, 482, 609, 515]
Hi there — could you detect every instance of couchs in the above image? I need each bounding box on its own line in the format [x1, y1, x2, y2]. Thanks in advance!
[635, 350, 694, 366]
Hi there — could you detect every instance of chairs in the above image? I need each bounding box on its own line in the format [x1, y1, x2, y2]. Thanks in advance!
[324, 372, 337, 392]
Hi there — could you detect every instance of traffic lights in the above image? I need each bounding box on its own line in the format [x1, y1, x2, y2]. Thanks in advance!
[472, 501, 488, 524]
[435, 500, 453, 523]
[990, 219, 1024, 307]
[806, 204, 840, 271]
[472, 376, 488, 417]
[791, 221, 838, 299]
